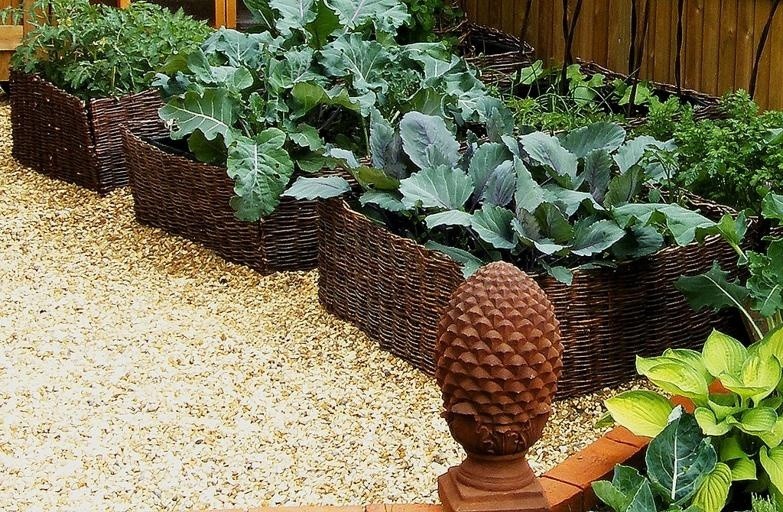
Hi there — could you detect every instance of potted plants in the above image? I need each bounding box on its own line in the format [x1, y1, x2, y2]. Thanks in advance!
[118, 0, 478, 279]
[0, 0, 219, 195]
[319, 109, 760, 404]
